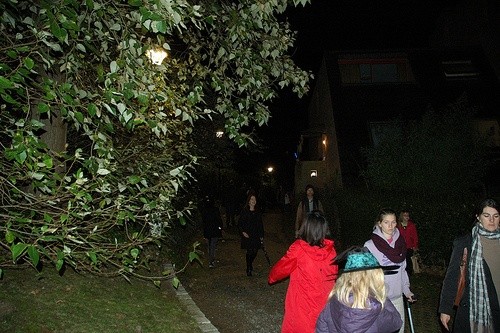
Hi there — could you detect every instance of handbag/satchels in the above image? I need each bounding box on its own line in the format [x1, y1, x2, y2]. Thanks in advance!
[453, 235, 467, 306]
[411, 250, 426, 274]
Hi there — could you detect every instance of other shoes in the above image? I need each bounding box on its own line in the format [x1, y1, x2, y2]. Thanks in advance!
[209, 261, 213, 267]
[246, 269, 253, 278]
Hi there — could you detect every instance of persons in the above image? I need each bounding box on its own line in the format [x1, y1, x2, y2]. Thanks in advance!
[222, 177, 241, 224]
[396, 210, 420, 275]
[201, 200, 223, 268]
[267, 210, 339, 333]
[238, 194, 264, 276]
[295, 184, 325, 238]
[363, 208, 417, 333]
[315, 246, 403, 333]
[439, 200, 500, 333]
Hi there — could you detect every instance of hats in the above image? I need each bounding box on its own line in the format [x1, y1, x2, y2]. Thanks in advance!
[327, 247, 401, 272]
